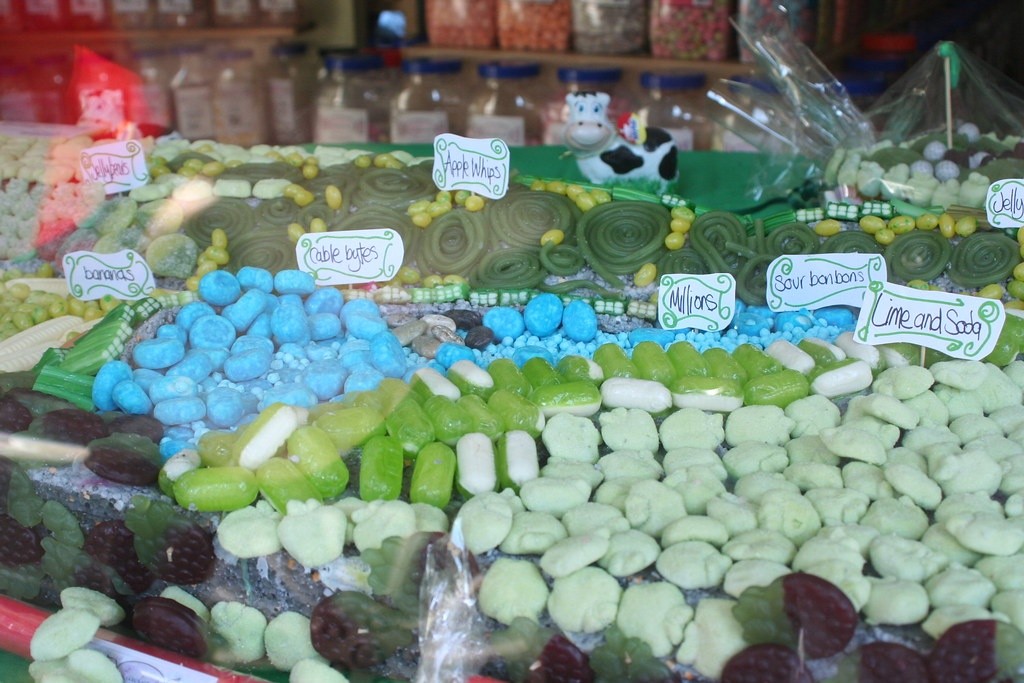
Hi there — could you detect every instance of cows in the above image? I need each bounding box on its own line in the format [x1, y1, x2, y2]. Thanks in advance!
[561, 91, 680, 193]
